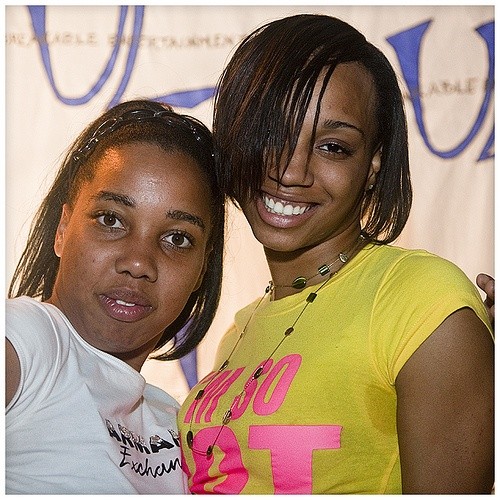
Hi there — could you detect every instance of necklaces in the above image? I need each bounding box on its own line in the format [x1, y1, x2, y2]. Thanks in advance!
[186, 229, 369, 456]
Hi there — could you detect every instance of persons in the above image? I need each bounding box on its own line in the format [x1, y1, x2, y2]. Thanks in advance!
[5, 96, 494, 494]
[176, 13, 495, 495]
